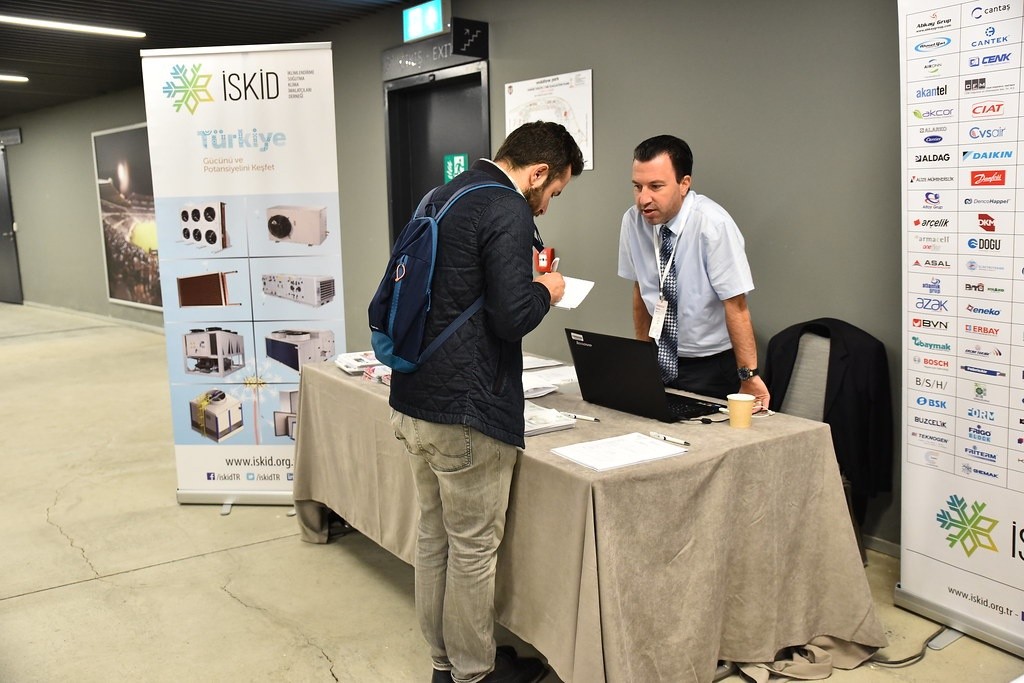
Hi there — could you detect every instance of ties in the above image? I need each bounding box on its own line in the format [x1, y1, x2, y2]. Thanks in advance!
[657, 226, 679, 384]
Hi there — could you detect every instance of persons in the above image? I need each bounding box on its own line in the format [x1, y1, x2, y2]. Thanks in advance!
[618, 134, 770, 414]
[389, 123, 584, 683]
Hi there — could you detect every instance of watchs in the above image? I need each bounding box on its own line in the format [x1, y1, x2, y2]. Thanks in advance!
[737, 367, 759, 381]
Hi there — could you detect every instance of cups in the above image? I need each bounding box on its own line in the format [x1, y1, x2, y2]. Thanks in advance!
[726, 394, 756, 429]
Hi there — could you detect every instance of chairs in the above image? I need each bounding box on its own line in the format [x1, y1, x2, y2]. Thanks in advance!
[766, 317, 892, 543]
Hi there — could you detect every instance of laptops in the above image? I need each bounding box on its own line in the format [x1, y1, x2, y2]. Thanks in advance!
[565, 328, 729, 423]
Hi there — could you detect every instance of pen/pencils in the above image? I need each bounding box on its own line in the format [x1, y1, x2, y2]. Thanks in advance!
[650, 431, 692, 446]
[562, 411, 602, 423]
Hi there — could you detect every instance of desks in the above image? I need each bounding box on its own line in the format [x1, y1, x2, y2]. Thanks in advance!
[299, 350, 890, 683]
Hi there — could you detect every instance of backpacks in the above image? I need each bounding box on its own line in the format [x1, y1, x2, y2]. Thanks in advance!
[366, 180, 520, 373]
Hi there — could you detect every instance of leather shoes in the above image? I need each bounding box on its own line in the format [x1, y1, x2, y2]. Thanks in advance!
[433, 645, 553, 683]
